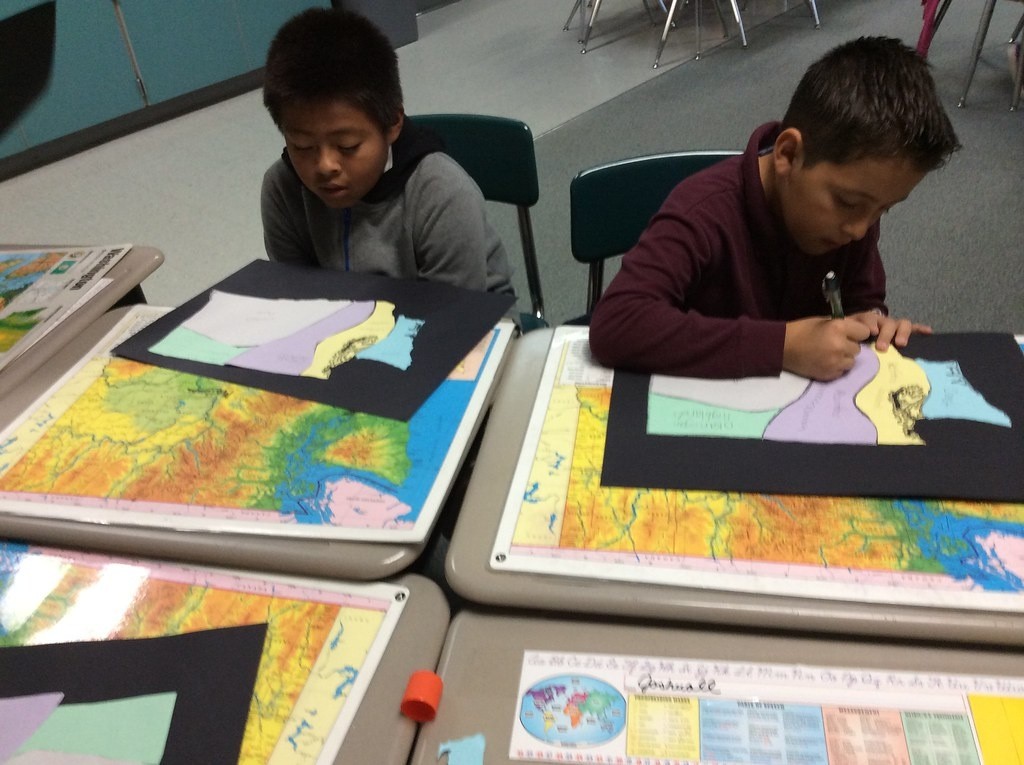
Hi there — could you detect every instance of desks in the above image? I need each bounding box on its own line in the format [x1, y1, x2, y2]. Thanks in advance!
[447, 321, 1024, 657]
[0, 242, 165, 403]
[0, 307, 520, 582]
[406, 606, 1024, 765]
[98, 540, 451, 765]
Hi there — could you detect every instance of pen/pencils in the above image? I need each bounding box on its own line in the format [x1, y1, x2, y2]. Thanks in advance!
[821, 271, 846, 319]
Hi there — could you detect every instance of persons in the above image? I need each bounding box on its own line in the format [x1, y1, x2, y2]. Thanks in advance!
[590, 37, 963, 380]
[262, 8, 515, 303]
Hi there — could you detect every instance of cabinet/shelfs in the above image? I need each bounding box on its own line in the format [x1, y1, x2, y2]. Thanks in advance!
[0, 0, 334, 184]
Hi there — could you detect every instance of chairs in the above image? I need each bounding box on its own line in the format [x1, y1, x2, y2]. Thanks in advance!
[392, 112, 551, 332]
[559, 151, 748, 327]
